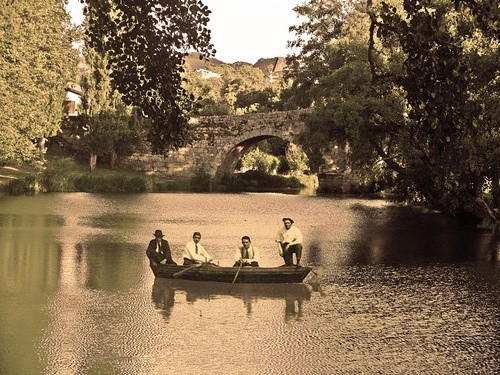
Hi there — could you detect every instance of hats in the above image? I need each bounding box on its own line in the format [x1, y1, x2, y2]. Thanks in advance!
[153, 229, 165, 237]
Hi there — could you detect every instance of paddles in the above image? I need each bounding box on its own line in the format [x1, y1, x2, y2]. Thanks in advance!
[173, 260, 211, 279]
[228, 262, 242, 294]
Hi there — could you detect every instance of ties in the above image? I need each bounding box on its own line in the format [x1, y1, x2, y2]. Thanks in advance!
[196, 244, 198, 254]
[158, 241, 161, 254]
[246, 248, 249, 259]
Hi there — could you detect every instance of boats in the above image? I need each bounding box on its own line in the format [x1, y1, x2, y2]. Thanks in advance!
[150, 263, 312, 284]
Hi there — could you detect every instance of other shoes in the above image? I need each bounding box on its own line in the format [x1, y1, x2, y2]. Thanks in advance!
[297, 262, 302, 267]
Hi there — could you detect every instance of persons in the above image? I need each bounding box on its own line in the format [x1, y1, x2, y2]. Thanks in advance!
[146, 230, 178, 265]
[183, 231, 214, 266]
[275, 218, 303, 267]
[233, 236, 260, 267]
